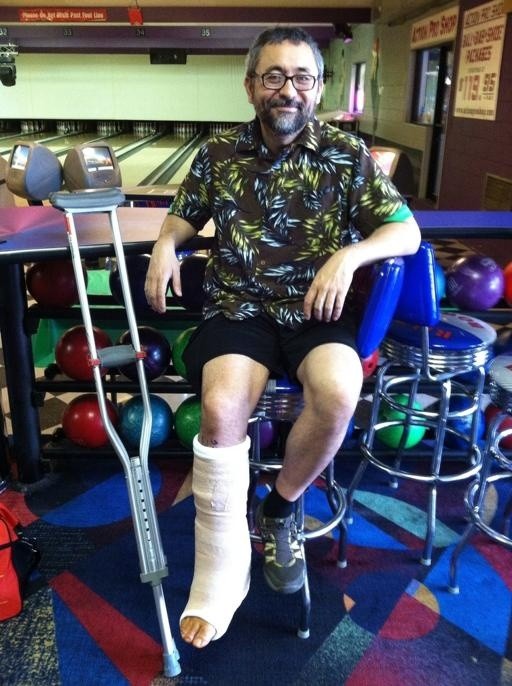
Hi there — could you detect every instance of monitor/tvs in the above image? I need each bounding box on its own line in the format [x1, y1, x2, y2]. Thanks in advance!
[369, 146, 414, 194]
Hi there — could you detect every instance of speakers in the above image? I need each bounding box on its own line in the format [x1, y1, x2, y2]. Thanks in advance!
[150, 48, 187, 64]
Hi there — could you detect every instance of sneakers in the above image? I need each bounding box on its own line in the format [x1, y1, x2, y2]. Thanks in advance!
[252, 491, 308, 596]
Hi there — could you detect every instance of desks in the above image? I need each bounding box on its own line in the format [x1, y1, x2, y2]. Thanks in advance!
[0, 206, 215, 483]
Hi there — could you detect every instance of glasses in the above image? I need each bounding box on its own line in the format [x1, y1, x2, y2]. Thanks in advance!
[249, 68, 322, 92]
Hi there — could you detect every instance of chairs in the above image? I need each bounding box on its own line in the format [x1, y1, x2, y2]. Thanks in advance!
[161, 233, 405, 641]
[448, 351, 512, 595]
[335, 252, 497, 567]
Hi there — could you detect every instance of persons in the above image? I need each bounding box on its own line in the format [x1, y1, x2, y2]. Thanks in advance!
[140, 26, 424, 655]
